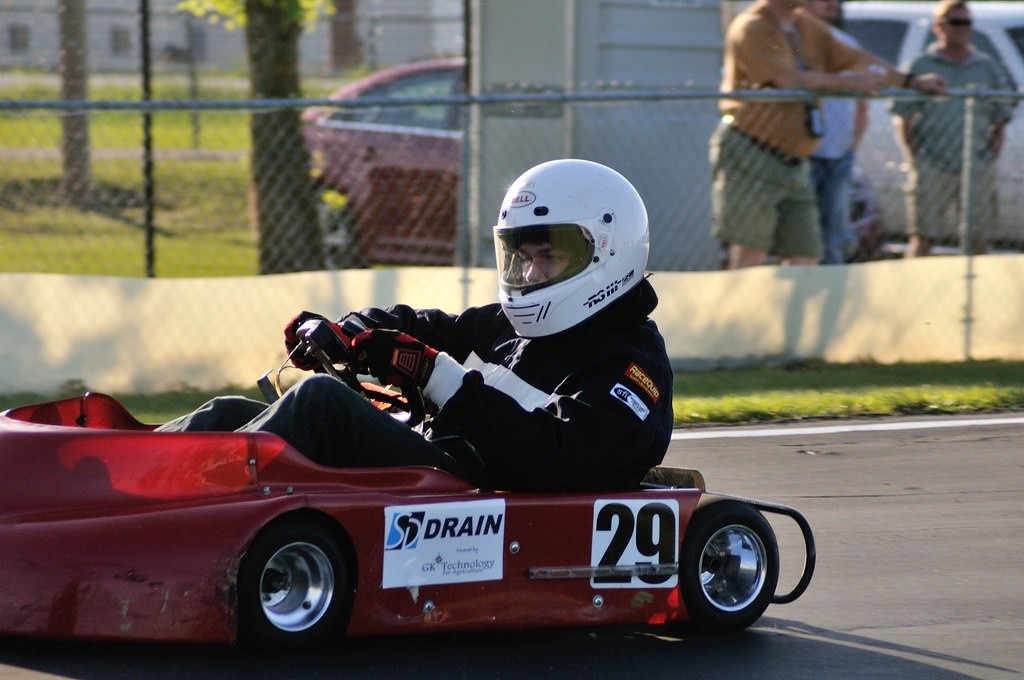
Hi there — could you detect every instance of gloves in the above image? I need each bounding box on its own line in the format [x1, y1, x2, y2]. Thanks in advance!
[284, 312, 354, 371]
[350, 328, 439, 393]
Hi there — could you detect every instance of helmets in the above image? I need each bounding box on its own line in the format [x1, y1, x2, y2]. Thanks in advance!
[492, 157, 650, 338]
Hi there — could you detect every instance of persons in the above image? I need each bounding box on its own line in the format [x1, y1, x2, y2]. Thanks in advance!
[707, 0, 945, 271]
[888, 0, 1024, 259]
[153, 159, 674, 495]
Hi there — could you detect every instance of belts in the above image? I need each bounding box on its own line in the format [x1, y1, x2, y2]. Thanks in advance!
[729, 124, 804, 167]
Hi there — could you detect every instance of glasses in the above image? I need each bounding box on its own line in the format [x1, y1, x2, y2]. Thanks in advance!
[941, 18, 971, 27]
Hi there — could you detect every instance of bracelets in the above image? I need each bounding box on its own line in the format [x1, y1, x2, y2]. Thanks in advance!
[901, 72, 914, 88]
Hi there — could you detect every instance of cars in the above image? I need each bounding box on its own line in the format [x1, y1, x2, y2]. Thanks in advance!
[242, 56, 468, 269]
[837, 0, 1024, 256]
